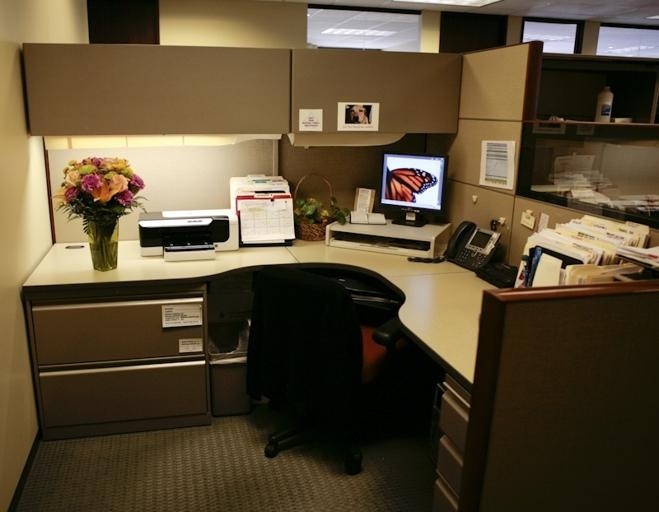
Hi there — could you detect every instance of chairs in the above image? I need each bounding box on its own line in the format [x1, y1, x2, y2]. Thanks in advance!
[245, 272, 405, 472]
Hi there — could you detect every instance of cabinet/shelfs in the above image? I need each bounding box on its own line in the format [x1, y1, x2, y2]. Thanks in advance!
[32, 291, 209, 428]
[536, 71, 659, 220]
[431, 375, 472, 511]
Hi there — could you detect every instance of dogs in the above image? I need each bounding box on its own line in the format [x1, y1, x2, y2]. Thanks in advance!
[346, 105, 368, 124]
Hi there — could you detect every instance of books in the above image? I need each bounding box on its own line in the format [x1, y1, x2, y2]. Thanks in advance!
[515, 216, 659, 288]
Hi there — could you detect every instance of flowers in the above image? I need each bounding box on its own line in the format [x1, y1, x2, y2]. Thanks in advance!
[54, 158, 144, 270]
[294, 197, 350, 225]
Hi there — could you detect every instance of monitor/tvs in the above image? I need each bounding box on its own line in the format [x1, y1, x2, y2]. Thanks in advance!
[379, 151, 449, 227]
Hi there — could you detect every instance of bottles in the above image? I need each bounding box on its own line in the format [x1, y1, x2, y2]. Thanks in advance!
[593, 86, 614, 122]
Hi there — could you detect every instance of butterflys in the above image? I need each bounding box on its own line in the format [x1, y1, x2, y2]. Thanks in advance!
[384, 167, 438, 203]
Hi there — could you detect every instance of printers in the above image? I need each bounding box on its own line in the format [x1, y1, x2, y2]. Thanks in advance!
[138, 209, 239, 261]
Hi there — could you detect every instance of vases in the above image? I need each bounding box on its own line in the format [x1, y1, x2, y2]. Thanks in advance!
[87, 219, 119, 270]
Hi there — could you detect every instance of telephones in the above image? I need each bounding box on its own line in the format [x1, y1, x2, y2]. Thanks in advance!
[443, 221, 502, 271]
